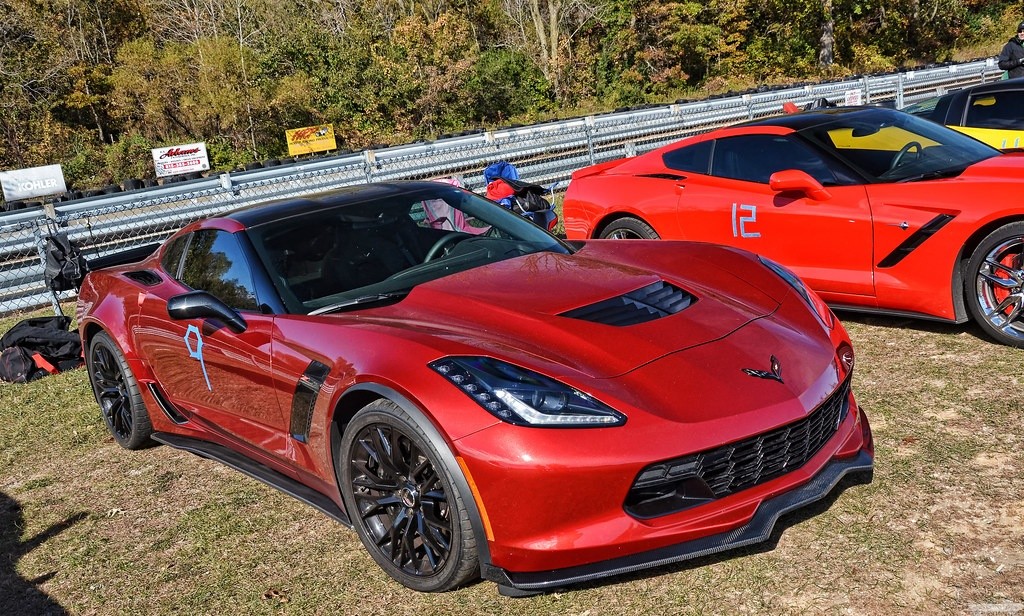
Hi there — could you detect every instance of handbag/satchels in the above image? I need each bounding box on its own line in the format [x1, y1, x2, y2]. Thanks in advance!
[484, 177, 551, 213]
[43, 231, 90, 291]
[0, 316, 84, 384]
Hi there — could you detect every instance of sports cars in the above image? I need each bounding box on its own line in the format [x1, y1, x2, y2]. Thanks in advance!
[74, 179, 879, 596]
[827, 73, 1024, 152]
[560, 104, 1023, 351]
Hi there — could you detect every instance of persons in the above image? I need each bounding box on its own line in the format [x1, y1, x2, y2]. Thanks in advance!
[997, 21, 1024, 78]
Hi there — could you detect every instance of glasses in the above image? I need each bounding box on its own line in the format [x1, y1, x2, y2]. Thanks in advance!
[1017, 30, 1024, 33]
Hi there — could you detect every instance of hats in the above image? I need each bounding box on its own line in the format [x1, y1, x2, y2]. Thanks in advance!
[1018, 22, 1024, 29]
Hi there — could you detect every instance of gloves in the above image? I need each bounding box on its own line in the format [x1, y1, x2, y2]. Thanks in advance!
[1019, 57, 1024, 65]
